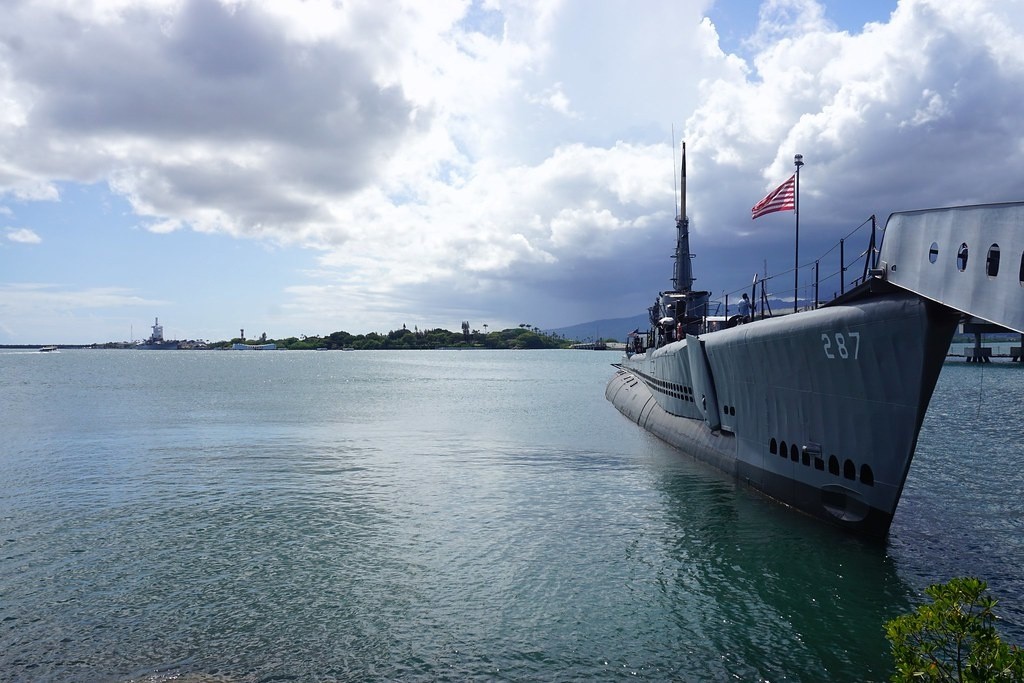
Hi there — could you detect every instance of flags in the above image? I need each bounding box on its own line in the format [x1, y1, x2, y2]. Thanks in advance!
[752, 174, 795, 219]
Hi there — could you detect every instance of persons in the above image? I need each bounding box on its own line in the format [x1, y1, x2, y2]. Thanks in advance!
[632, 336, 643, 352]
[661, 334, 666, 345]
[677, 319, 682, 341]
[736, 315, 750, 325]
[738, 293, 752, 315]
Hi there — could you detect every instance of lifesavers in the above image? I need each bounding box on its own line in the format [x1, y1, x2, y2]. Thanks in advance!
[677, 323, 682, 341]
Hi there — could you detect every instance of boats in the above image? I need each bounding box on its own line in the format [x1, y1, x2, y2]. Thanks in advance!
[605, 123, 1024, 542]
[317, 348, 327, 351]
[39, 344, 57, 351]
[232, 343, 277, 350]
[343, 348, 354, 351]
[135, 317, 178, 350]
